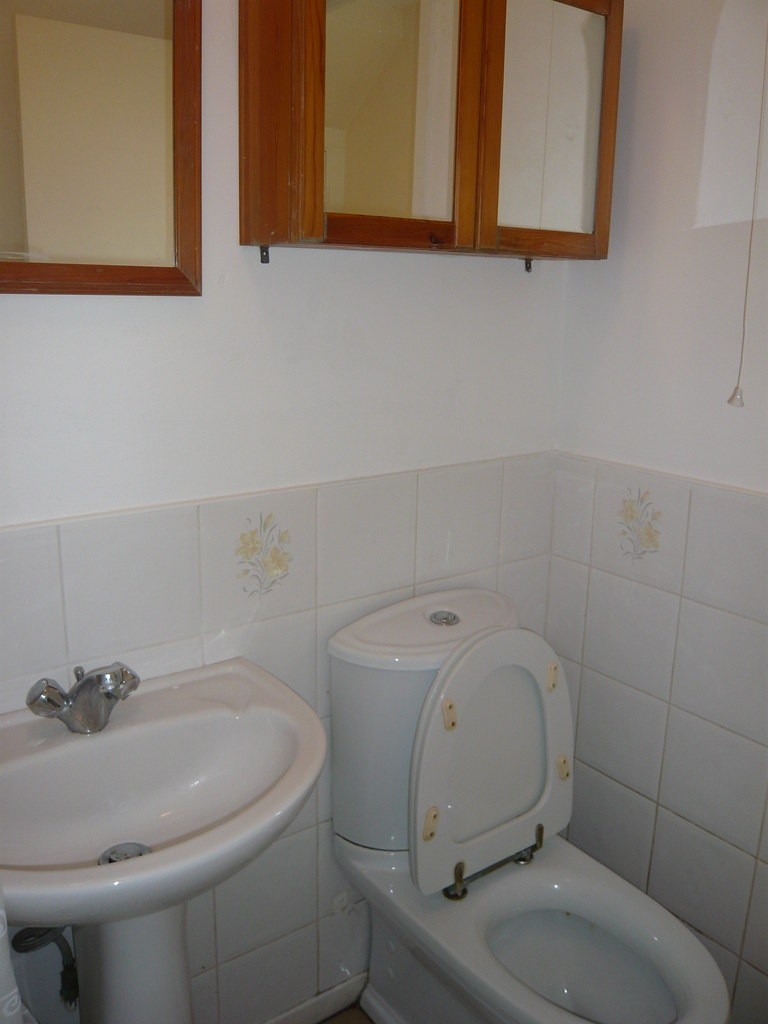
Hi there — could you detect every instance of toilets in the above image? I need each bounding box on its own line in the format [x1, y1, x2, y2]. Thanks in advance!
[324, 584, 734, 1023]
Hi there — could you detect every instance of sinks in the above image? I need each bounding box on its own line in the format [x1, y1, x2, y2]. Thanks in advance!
[0, 635, 314, 931]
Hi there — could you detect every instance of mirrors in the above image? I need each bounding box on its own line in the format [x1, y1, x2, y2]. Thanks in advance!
[0, 0, 203, 297]
[496, 0, 608, 234]
[323, 0, 461, 223]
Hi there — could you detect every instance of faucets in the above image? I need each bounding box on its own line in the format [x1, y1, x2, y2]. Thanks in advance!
[24, 659, 142, 735]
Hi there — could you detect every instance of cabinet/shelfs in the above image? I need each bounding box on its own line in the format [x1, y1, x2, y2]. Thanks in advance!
[238, 0, 624, 260]
